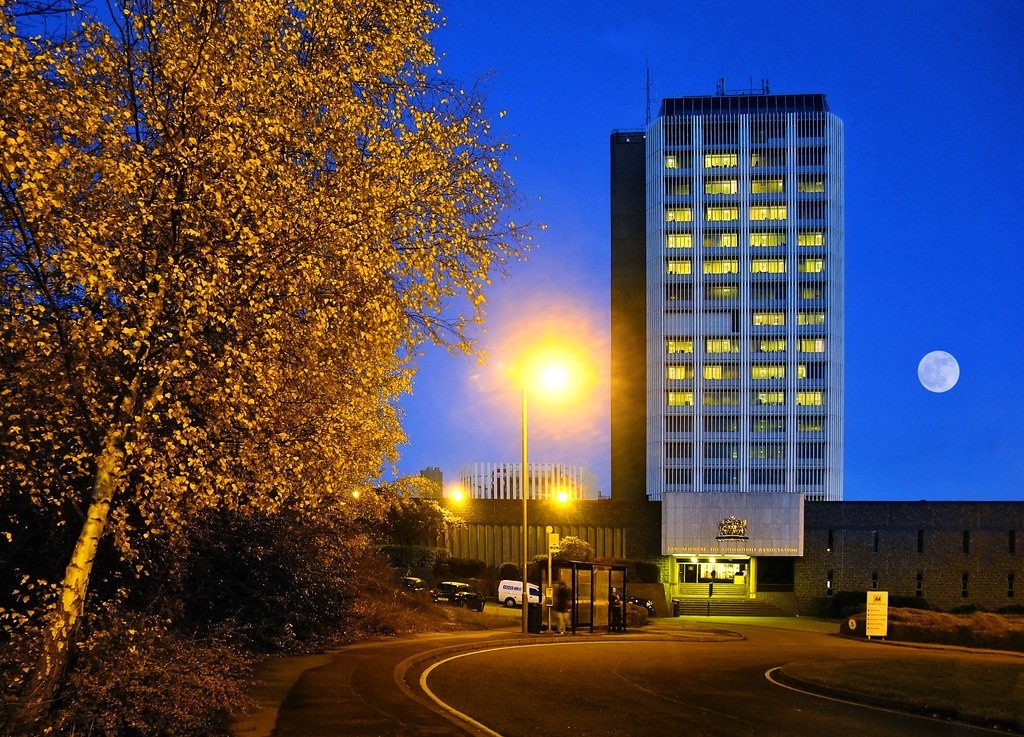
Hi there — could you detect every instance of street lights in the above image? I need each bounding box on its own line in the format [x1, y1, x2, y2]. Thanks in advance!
[521, 350, 570, 637]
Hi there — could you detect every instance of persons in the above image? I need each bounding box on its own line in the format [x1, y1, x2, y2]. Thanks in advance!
[555, 580, 572, 634]
[611, 586, 621, 633]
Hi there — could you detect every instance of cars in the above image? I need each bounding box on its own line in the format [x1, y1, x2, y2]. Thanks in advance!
[435, 582, 485, 609]
[400, 577, 421, 593]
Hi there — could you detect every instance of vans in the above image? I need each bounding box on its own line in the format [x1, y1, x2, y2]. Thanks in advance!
[498, 580, 545, 608]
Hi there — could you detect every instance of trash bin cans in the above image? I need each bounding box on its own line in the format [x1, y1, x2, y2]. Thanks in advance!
[528, 603, 542, 634]
[670, 599, 680, 617]
